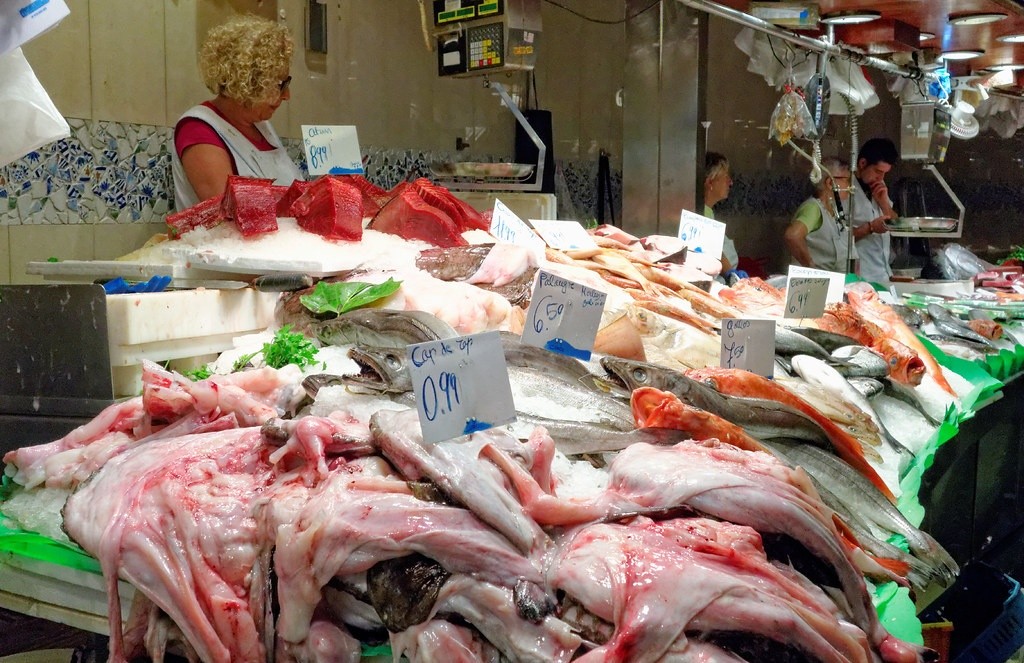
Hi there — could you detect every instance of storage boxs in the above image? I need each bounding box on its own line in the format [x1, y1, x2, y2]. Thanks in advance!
[891, 277, 973, 296]
[892, 267, 922, 278]
[917, 552, 1024, 662]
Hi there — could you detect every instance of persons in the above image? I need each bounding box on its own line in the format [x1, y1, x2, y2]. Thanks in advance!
[702, 150, 747, 287]
[785, 157, 858, 273]
[171, 12, 305, 213]
[836, 137, 898, 284]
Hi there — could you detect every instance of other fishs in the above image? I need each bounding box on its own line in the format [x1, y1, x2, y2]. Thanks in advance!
[923, 302, 1006, 355]
[592, 355, 965, 594]
[773, 287, 960, 460]
[313, 307, 675, 458]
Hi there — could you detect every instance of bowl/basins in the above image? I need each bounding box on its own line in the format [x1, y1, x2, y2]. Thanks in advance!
[892, 268, 922, 278]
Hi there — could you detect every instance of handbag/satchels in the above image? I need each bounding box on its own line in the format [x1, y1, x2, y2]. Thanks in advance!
[0, 47, 71, 173]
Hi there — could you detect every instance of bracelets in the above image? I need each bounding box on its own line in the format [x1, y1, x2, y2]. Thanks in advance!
[869, 221, 873, 235]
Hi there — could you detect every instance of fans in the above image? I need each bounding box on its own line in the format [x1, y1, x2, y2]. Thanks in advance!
[944, 76, 982, 140]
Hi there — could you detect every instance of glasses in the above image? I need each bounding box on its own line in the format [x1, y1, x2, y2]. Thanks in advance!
[278, 76, 291, 92]
